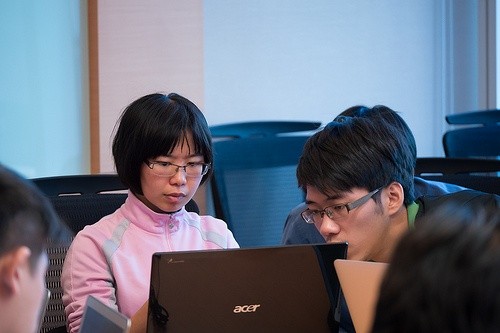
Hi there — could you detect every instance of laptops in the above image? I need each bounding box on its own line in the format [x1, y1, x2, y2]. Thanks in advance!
[146, 242, 348, 333]
[78, 294, 132, 333]
[334, 259, 389, 333]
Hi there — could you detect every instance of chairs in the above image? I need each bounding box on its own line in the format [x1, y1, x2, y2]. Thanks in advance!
[27, 174, 199, 333]
[209, 110, 322, 248]
[414, 109, 500, 198]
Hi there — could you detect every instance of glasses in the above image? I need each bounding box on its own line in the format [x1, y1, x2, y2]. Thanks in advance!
[141, 158, 213, 177]
[300, 184, 387, 224]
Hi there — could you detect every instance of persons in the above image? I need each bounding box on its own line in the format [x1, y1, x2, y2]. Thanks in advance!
[60, 93, 240, 333]
[296, 115, 441, 333]
[0, 163, 52, 333]
[283, 104, 474, 245]
[368, 190, 500, 333]
